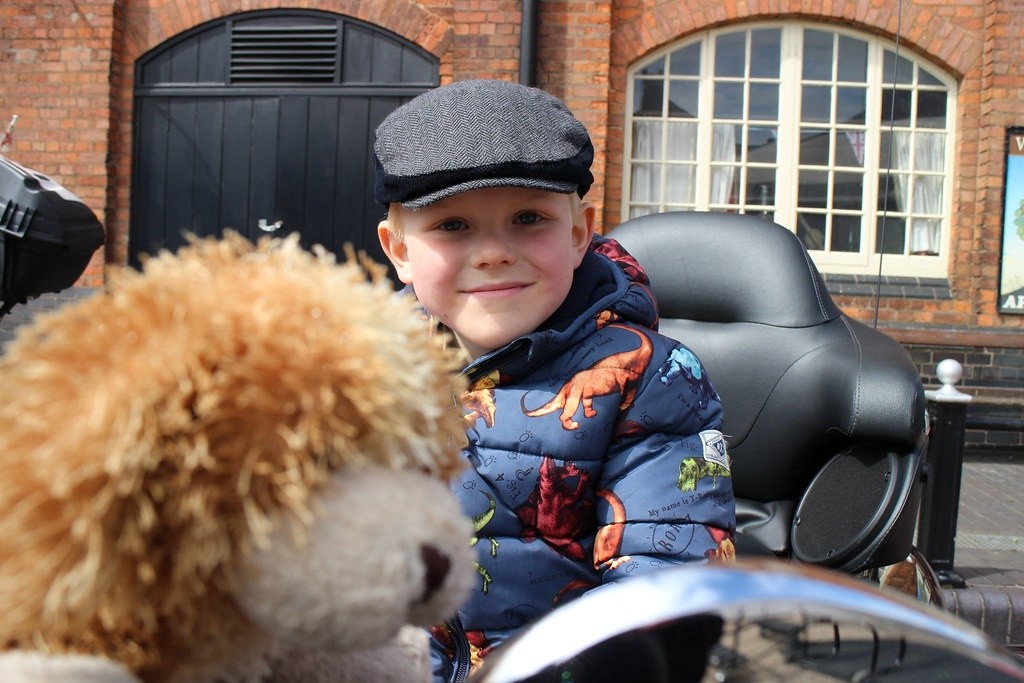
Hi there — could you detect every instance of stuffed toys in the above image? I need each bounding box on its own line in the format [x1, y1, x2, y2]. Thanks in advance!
[0, 227, 477, 682]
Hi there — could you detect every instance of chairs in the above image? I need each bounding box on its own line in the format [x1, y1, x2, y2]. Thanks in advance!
[601, 212, 926, 573]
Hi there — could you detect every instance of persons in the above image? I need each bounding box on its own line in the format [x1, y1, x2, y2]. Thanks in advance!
[372, 80, 734, 683]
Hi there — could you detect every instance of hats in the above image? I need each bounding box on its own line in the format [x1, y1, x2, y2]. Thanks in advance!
[372, 78, 596, 211]
